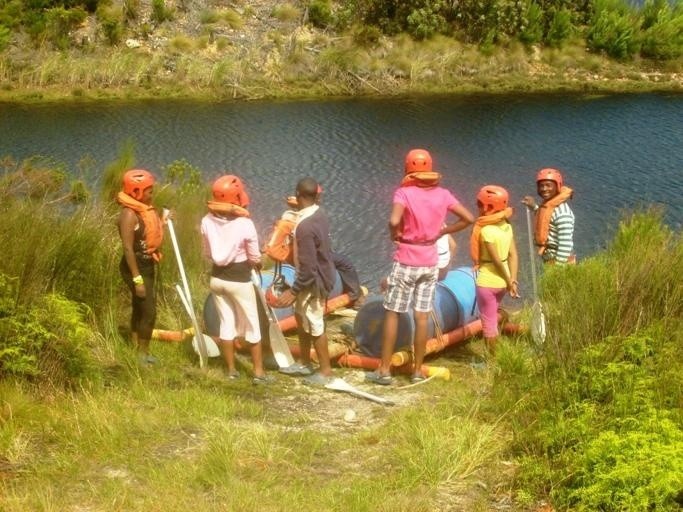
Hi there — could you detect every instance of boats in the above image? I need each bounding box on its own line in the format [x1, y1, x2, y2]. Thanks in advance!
[184, 265, 369, 363]
[311, 263, 529, 381]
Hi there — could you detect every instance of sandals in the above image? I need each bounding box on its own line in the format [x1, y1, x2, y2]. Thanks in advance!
[366, 370, 393, 384]
[253, 376, 274, 383]
[410, 372, 425, 382]
[280, 364, 312, 374]
[303, 374, 333, 384]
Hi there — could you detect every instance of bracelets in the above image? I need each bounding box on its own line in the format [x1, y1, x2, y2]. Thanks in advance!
[533, 204, 540, 212]
[133, 275, 144, 287]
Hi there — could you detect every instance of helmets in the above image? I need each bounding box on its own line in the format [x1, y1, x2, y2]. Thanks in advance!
[476, 186, 509, 214]
[213, 175, 249, 207]
[405, 149, 432, 173]
[123, 170, 154, 201]
[266, 280, 293, 307]
[536, 168, 562, 193]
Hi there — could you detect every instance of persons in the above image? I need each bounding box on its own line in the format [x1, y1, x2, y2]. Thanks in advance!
[379, 221, 458, 293]
[275, 176, 338, 387]
[199, 173, 280, 388]
[472, 184, 522, 361]
[266, 184, 373, 312]
[521, 168, 580, 277]
[362, 145, 477, 387]
[117, 167, 178, 358]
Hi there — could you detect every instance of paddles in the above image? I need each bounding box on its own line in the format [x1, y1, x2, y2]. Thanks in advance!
[525, 205, 546, 346]
[250, 269, 295, 368]
[166, 214, 208, 369]
[324, 378, 403, 406]
